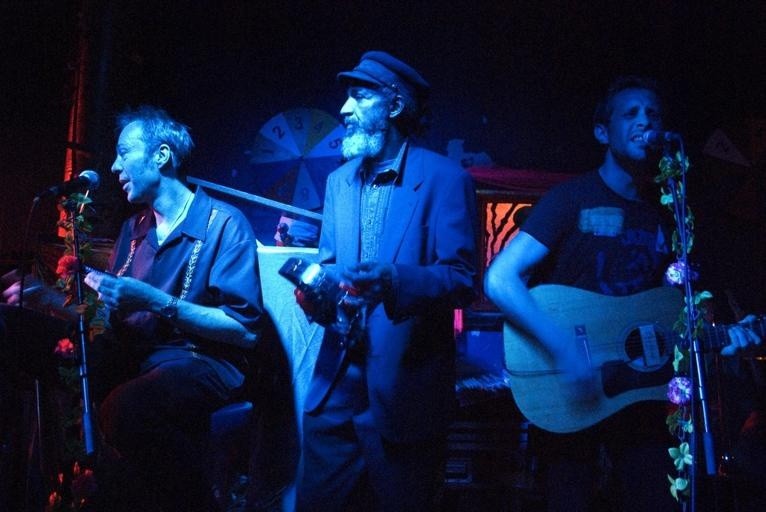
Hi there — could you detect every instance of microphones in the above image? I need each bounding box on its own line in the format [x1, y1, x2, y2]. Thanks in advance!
[644, 130, 674, 146]
[33, 170, 100, 200]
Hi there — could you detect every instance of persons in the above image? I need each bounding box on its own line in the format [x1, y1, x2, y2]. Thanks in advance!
[4, 107, 267, 512]
[484, 80, 765, 511]
[293, 52, 488, 510]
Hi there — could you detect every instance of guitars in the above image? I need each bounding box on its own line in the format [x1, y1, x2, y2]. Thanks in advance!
[503, 285, 765, 433]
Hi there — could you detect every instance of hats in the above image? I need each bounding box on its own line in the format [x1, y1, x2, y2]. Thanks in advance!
[337, 51, 430, 110]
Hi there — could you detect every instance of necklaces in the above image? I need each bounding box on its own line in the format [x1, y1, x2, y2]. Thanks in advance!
[168, 192, 191, 232]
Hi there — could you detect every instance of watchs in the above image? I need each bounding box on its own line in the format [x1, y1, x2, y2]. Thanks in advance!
[159, 297, 179, 319]
